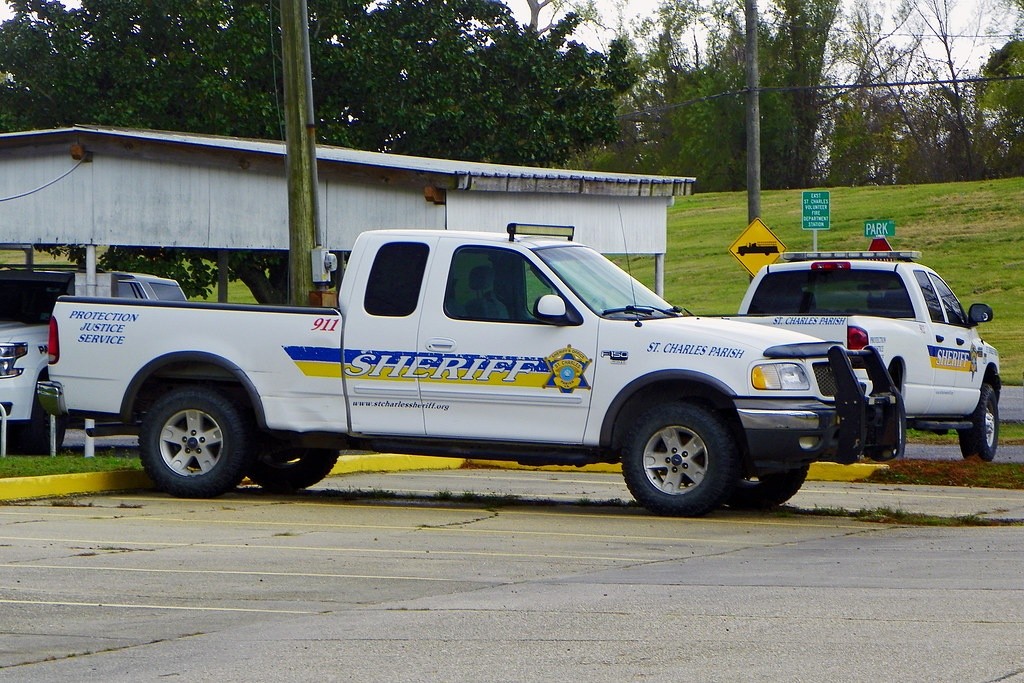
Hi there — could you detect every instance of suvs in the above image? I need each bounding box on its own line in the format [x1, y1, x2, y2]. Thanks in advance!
[0, 264, 190, 456]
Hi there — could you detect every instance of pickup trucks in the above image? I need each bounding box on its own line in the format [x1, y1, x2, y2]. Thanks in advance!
[711, 247, 1002, 459]
[36, 213, 905, 517]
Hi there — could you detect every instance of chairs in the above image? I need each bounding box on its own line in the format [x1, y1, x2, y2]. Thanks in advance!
[444, 272, 472, 320]
[463, 265, 511, 319]
[779, 286, 915, 318]
